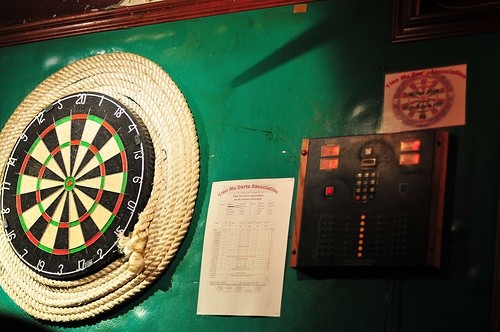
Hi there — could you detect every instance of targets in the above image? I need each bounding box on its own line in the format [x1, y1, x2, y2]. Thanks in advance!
[1, 91, 153, 280]
